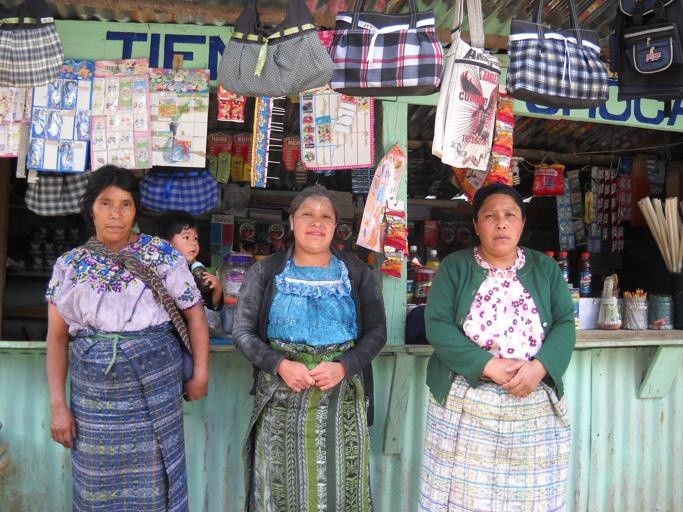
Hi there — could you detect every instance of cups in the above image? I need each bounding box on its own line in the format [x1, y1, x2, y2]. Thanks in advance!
[597, 295, 674, 331]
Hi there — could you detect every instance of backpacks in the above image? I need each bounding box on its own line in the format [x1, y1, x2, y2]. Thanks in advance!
[609, 0, 681, 101]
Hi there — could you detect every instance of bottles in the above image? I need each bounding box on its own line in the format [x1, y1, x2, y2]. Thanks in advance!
[545, 251, 555, 260]
[405, 244, 439, 304]
[556, 249, 570, 285]
[187, 257, 213, 294]
[578, 250, 593, 296]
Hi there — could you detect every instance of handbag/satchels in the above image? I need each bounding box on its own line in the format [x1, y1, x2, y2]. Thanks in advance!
[531, 153, 565, 197]
[217, 0, 334, 97]
[431, 0, 501, 173]
[506, 0, 609, 109]
[24, 168, 91, 217]
[137, 169, 218, 216]
[327, 0, 444, 96]
[0, 1, 64, 87]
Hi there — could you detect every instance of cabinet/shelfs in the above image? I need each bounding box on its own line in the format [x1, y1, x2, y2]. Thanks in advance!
[1, 181, 89, 319]
[206, 186, 557, 341]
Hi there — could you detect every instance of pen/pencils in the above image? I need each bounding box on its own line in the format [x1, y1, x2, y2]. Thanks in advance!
[623, 288, 647, 329]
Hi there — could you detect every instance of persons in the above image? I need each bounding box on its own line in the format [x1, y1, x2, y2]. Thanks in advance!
[417, 182, 576, 512]
[153, 210, 224, 328]
[45, 164, 209, 512]
[231, 185, 386, 512]
